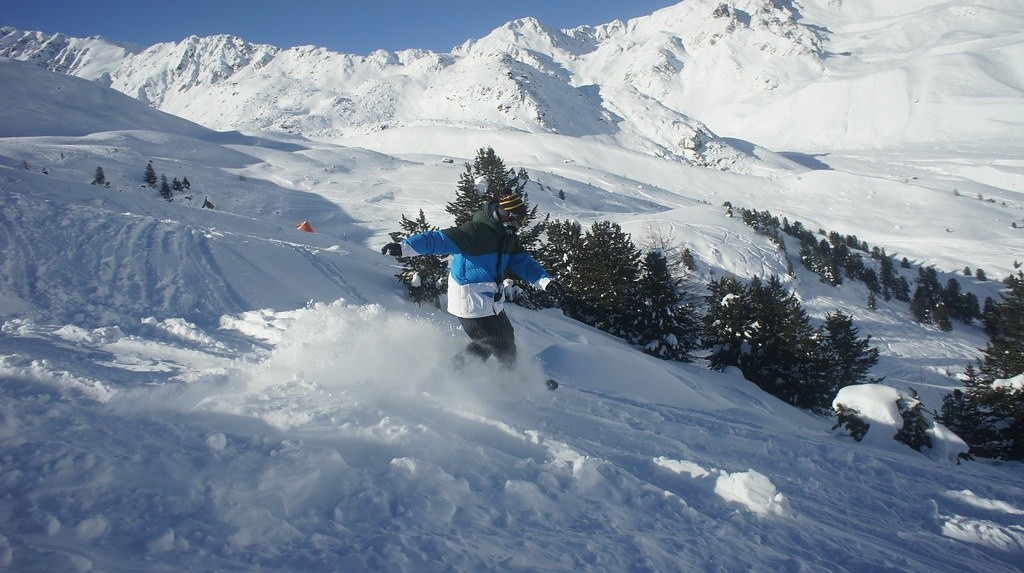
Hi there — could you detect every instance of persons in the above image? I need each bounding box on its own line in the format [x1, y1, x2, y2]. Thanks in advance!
[381, 188, 565, 371]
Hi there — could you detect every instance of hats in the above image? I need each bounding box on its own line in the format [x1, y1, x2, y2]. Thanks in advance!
[498, 188, 527, 219]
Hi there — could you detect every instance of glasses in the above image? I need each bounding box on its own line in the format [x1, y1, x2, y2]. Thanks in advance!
[516, 217, 526, 227]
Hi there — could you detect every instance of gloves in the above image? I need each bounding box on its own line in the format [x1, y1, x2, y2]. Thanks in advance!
[381, 242, 402, 256]
[546, 282, 565, 297]
